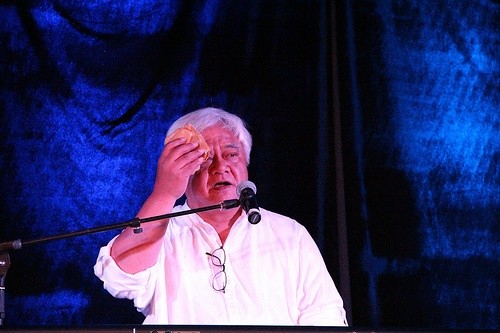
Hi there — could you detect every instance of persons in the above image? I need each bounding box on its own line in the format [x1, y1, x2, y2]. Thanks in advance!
[93, 107, 349, 326]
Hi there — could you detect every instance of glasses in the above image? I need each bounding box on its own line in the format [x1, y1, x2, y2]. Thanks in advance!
[206, 245, 227, 293]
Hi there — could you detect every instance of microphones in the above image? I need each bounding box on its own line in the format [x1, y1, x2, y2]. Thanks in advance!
[236, 181, 262, 224]
